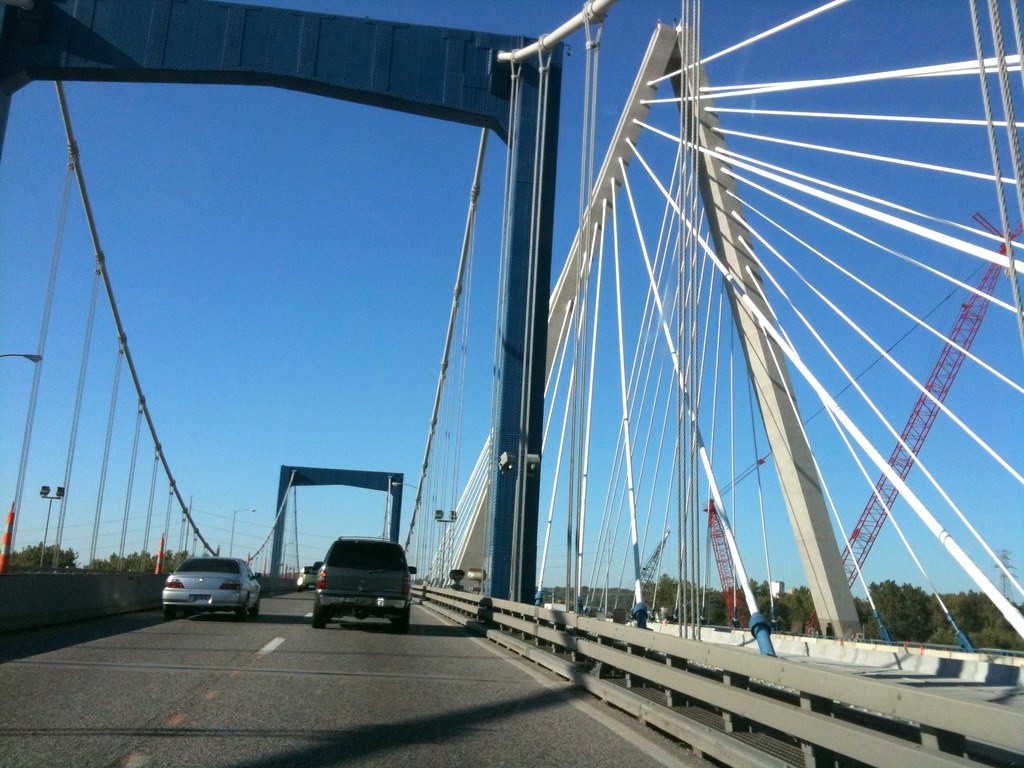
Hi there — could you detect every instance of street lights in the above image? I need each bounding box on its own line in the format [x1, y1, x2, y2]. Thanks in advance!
[281, 541, 300, 575]
[38, 486, 65, 569]
[230, 509, 256, 557]
[390, 482, 420, 580]
[435, 510, 458, 583]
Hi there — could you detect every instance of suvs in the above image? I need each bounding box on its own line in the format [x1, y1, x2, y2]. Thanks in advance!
[307, 536, 418, 634]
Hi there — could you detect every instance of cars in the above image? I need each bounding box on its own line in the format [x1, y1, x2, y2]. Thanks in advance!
[160, 556, 261, 622]
[296, 566, 319, 592]
